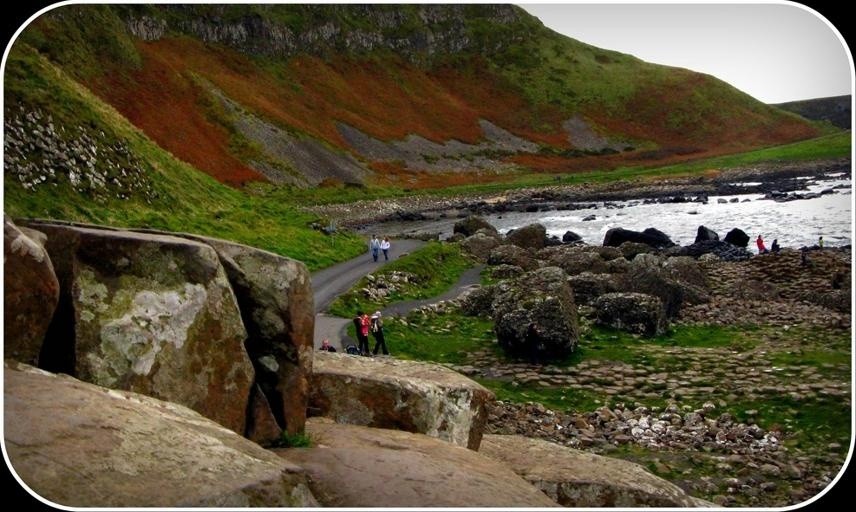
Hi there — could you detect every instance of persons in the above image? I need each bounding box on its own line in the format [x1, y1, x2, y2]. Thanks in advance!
[361, 316, 369, 336]
[380, 236, 391, 261]
[771, 238, 781, 254]
[353, 310, 370, 357]
[318, 339, 336, 352]
[819, 236, 824, 252]
[757, 235, 770, 253]
[370, 234, 381, 262]
[370, 311, 391, 356]
